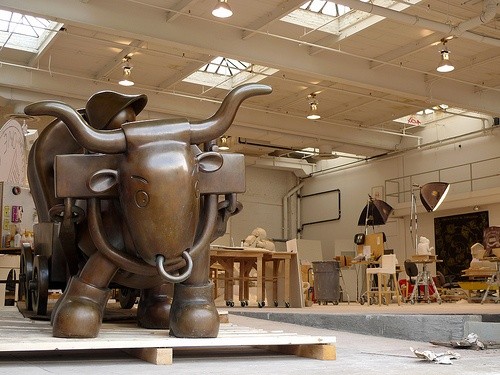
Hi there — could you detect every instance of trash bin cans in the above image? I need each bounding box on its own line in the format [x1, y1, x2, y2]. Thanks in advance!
[311, 261, 341, 305]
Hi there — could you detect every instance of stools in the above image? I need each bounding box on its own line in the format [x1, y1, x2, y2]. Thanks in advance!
[366, 254, 402, 307]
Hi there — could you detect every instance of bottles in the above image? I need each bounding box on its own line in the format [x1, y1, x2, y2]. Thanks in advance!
[4, 231, 34, 248]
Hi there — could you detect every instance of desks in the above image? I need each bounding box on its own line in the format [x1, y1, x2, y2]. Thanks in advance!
[207, 249, 297, 307]
[405, 260, 443, 305]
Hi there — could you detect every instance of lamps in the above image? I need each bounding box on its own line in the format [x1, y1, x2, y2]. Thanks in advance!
[304, 92, 322, 119]
[119, 56, 135, 87]
[212, 0, 234, 18]
[218, 135, 229, 151]
[436, 43, 454, 73]
